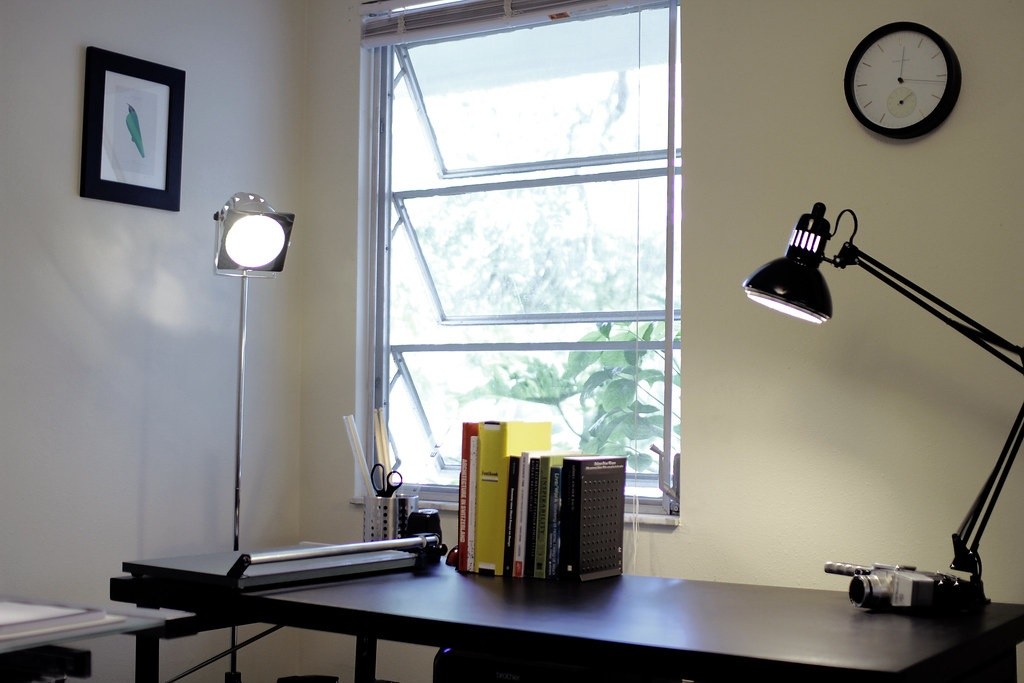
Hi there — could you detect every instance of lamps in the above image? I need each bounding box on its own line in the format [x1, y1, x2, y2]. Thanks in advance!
[744, 201, 1024, 621]
[212, 192, 295, 683]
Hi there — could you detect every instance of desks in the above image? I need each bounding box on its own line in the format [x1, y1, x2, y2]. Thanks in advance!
[110, 545, 1024, 683]
[0, 598, 165, 683]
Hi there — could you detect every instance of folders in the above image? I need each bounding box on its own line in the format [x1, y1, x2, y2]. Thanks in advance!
[475, 420, 553, 576]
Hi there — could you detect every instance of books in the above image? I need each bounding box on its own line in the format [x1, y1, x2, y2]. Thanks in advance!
[458, 421, 628, 583]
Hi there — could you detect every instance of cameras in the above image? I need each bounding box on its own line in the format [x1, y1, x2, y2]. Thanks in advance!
[824, 560, 918, 614]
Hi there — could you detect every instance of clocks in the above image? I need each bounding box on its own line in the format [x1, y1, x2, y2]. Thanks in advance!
[844, 21, 962, 139]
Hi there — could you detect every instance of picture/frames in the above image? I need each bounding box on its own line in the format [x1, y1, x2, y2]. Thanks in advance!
[79, 46, 186, 211]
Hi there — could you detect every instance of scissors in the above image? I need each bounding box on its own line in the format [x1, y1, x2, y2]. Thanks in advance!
[370, 463, 403, 537]
